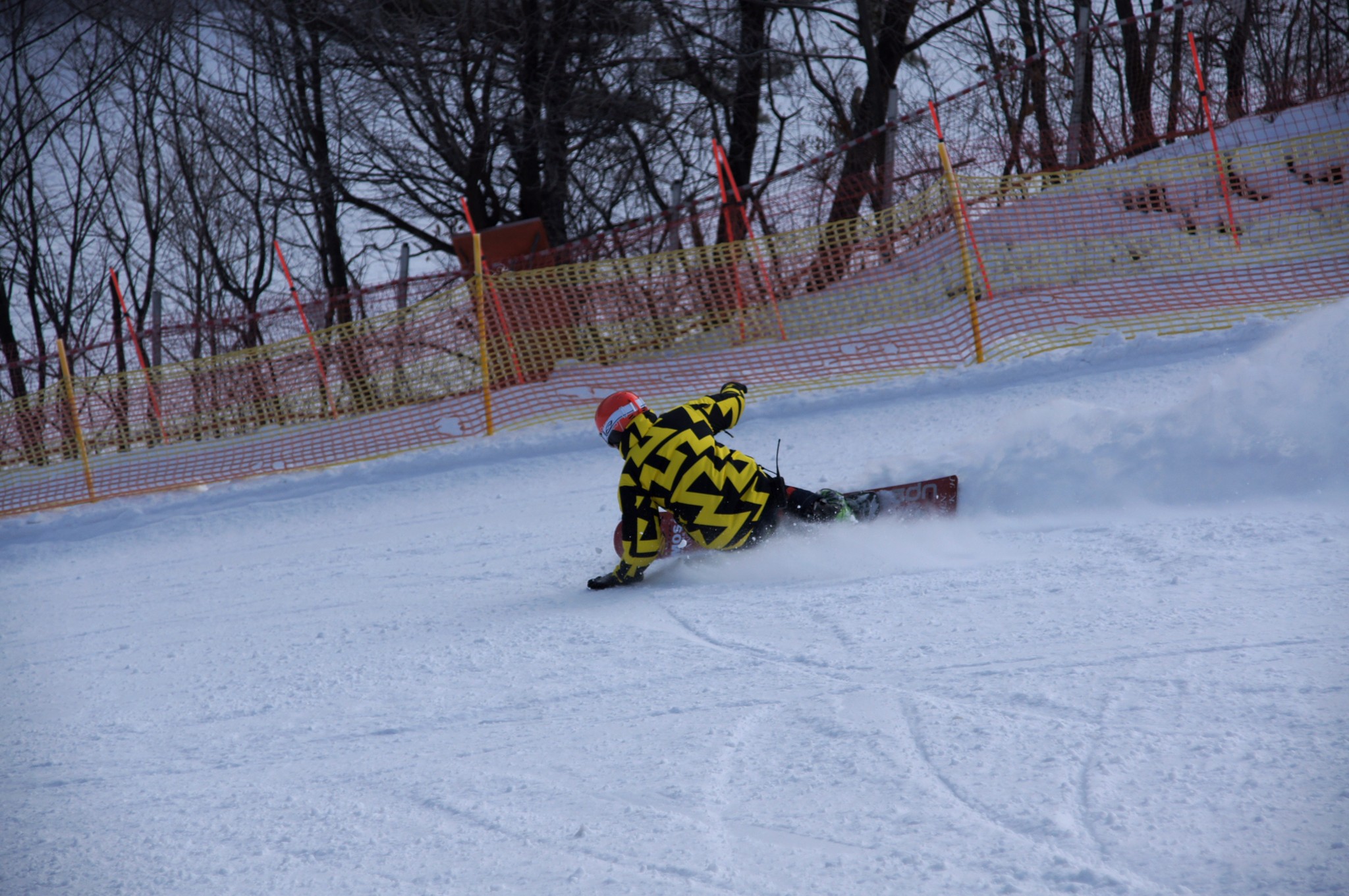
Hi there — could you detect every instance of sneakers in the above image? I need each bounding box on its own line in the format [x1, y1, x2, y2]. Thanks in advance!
[816, 488, 859, 519]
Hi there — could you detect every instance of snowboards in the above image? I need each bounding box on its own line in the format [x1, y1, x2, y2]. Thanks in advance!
[613, 476, 959, 561]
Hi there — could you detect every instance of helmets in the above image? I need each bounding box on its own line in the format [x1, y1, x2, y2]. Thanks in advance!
[595, 391, 649, 448]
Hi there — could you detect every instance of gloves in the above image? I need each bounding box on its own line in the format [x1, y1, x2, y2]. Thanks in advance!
[588, 572, 622, 591]
[725, 381, 747, 393]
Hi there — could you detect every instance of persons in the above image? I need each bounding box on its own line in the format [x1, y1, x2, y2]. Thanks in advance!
[582, 379, 893, 588]
